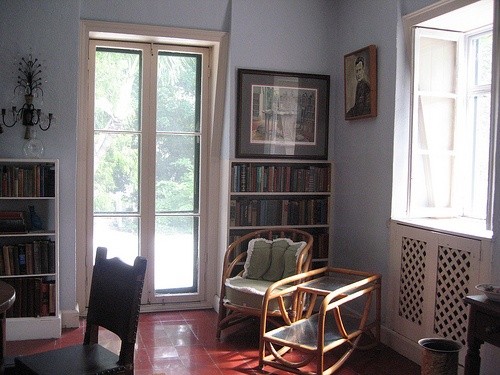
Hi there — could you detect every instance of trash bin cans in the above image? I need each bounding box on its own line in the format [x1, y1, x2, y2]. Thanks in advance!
[418, 337, 463, 375]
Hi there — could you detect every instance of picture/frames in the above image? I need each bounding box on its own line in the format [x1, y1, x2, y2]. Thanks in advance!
[236, 69, 330, 160]
[344, 45, 377, 120]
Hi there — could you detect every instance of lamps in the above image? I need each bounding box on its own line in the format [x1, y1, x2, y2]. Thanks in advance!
[0, 47, 55, 139]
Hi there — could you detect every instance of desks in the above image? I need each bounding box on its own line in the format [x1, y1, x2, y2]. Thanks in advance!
[464, 294, 500, 375]
[0, 281, 16, 375]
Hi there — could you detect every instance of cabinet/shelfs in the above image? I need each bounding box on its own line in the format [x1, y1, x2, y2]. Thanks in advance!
[258, 267, 381, 375]
[0, 159, 62, 341]
[227, 159, 333, 297]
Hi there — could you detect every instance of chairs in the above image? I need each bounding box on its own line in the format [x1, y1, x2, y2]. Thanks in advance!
[13, 247, 147, 375]
[215, 228, 314, 353]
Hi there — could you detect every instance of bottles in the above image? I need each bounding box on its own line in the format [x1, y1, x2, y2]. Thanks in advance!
[28, 206, 41, 229]
[23, 129, 45, 159]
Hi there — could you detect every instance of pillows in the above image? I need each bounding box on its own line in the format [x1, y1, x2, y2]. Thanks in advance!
[243, 238, 307, 285]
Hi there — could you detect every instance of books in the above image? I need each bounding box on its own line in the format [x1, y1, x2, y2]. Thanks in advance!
[228, 165, 329, 263]
[0, 164, 55, 198]
[0, 240, 56, 276]
[0, 211, 30, 234]
[0, 279, 56, 318]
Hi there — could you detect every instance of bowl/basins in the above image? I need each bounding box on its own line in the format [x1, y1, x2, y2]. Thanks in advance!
[475, 283, 500, 302]
